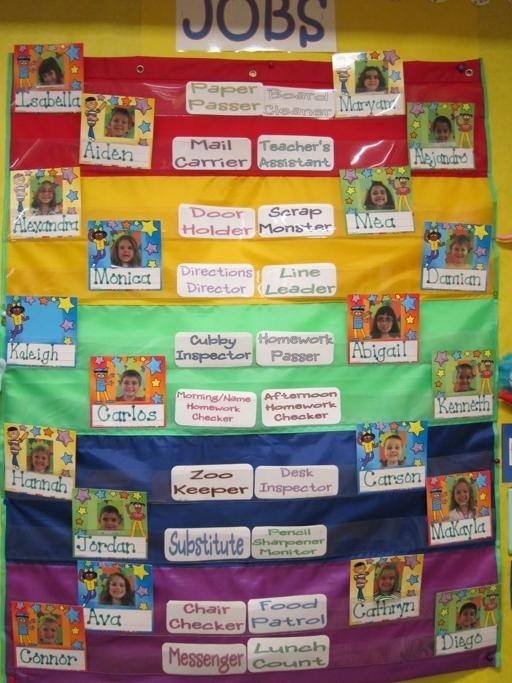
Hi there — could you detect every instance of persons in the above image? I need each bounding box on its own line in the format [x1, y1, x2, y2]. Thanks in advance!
[97, 502, 124, 530]
[103, 106, 134, 138]
[30, 49, 67, 86]
[111, 232, 141, 268]
[380, 433, 406, 468]
[37, 614, 62, 647]
[447, 233, 471, 269]
[373, 565, 403, 601]
[456, 603, 479, 632]
[118, 366, 146, 403]
[98, 571, 133, 605]
[429, 115, 453, 143]
[26, 180, 63, 212]
[447, 476, 477, 520]
[351, 60, 385, 93]
[26, 439, 52, 473]
[366, 303, 400, 339]
[360, 179, 395, 213]
[452, 362, 477, 393]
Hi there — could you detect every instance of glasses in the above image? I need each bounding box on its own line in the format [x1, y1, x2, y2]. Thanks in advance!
[376, 316, 393, 323]
[42, 627, 57, 632]
[39, 186, 54, 194]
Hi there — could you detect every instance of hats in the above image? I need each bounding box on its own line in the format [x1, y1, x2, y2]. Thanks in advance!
[427, 231, 441, 240]
[9, 302, 24, 314]
[82, 567, 98, 579]
